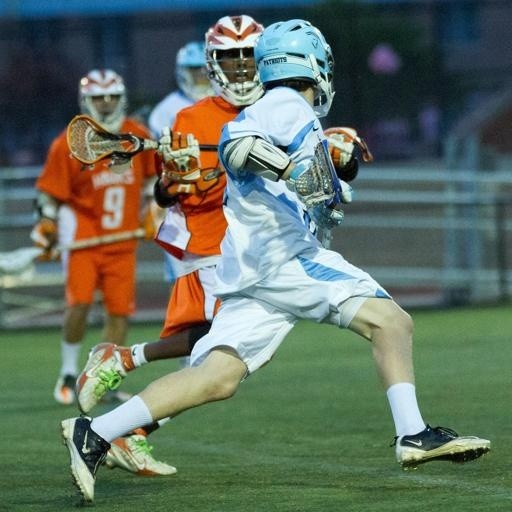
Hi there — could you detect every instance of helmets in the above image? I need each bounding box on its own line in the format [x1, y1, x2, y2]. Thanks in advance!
[203, 14, 266, 108]
[77, 67, 128, 135]
[253, 18, 336, 119]
[174, 41, 219, 102]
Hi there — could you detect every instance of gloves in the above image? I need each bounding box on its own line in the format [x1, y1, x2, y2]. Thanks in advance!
[139, 198, 167, 242]
[29, 218, 62, 263]
[157, 125, 202, 196]
[322, 126, 361, 169]
[285, 158, 354, 231]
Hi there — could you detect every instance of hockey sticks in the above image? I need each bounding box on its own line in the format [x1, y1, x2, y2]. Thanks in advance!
[65, 117, 219, 164]
[0, 230, 148, 285]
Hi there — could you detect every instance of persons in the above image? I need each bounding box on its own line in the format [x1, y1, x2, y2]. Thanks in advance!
[75, 14, 372, 476]
[60, 19, 491, 503]
[29, 67, 158, 405]
[147, 40, 215, 137]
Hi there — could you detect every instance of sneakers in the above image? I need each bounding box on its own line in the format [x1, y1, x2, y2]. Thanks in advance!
[388, 423, 494, 472]
[53, 374, 78, 405]
[100, 430, 178, 477]
[75, 341, 132, 415]
[59, 415, 112, 504]
